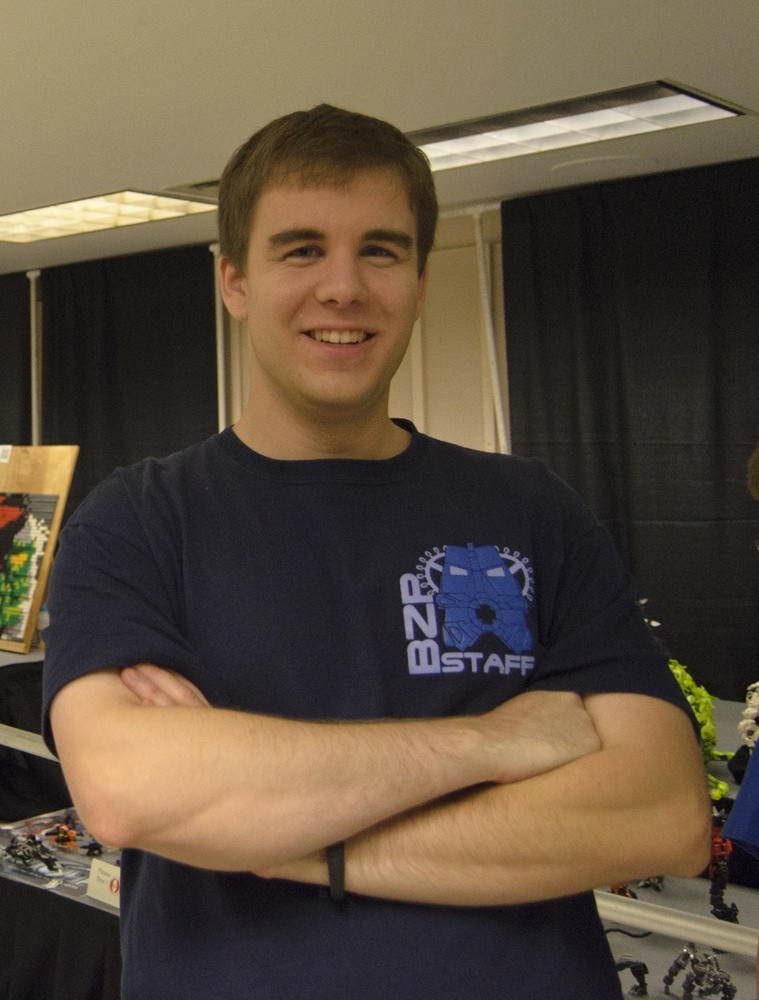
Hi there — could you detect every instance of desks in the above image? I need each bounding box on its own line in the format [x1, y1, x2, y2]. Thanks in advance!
[0, 806, 121, 1000]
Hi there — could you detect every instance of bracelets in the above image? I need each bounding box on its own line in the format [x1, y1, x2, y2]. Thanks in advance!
[326, 841, 346, 902]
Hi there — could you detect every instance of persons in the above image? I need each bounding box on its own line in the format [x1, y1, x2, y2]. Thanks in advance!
[39, 103, 714, 1000]
